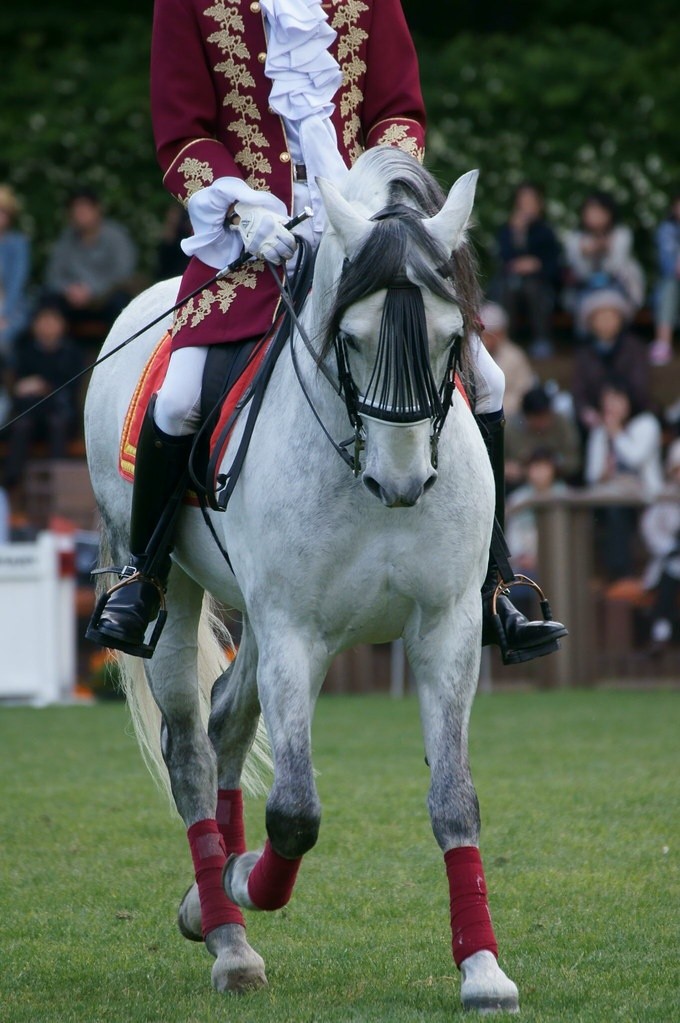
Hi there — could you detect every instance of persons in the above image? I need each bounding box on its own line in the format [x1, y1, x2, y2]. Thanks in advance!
[96, 1, 567, 649]
[1, 181, 680, 666]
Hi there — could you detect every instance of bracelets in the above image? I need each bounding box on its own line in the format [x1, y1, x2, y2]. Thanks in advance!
[229, 208, 239, 222]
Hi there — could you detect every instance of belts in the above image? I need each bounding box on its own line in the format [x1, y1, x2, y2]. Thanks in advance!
[293, 163, 307, 183]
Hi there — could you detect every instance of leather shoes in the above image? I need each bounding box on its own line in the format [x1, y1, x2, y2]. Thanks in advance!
[92, 562, 157, 644]
[481, 593, 569, 649]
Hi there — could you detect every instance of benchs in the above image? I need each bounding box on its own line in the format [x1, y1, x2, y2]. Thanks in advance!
[2, 306, 672, 681]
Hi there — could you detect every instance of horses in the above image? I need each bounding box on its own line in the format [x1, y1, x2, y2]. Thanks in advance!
[83, 143, 525, 1016]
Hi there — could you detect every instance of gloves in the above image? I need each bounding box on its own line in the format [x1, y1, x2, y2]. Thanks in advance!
[228, 204, 298, 266]
